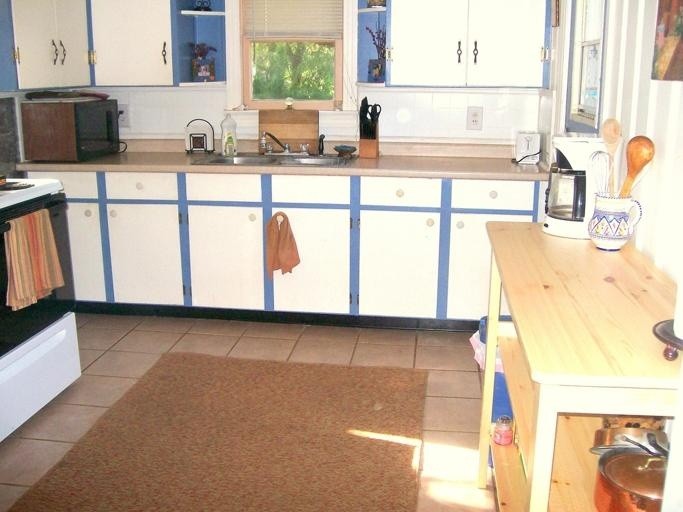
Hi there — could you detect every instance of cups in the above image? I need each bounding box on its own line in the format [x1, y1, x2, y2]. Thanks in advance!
[241, 104, 248, 111]
[587, 191, 643, 252]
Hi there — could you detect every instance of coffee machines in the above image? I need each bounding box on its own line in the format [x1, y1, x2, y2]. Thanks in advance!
[541, 136, 624, 240]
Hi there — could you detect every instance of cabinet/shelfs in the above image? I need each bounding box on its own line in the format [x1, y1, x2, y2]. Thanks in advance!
[88, 0, 233, 87]
[184, 171, 356, 317]
[26, 171, 192, 316]
[0, 1, 94, 92]
[355, 175, 539, 324]
[350, 0, 551, 88]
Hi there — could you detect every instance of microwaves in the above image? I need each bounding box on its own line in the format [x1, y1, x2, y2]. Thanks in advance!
[20, 99, 120, 162]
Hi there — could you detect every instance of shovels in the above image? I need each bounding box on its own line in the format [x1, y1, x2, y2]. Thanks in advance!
[623, 134, 654, 199]
[599, 117, 621, 199]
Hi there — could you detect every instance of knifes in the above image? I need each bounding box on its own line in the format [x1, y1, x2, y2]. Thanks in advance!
[360, 96, 381, 139]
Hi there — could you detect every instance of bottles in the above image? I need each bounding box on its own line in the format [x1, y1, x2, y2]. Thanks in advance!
[493, 415, 513, 445]
[225, 138, 234, 157]
[259, 131, 266, 154]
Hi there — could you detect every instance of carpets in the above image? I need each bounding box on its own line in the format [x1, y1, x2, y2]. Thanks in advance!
[8, 350, 430, 512]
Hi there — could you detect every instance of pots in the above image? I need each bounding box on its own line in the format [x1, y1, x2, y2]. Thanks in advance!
[592, 446, 668, 512]
[589, 428, 669, 457]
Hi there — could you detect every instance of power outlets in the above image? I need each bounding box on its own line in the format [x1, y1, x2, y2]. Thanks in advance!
[117, 104, 130, 128]
[465, 106, 483, 130]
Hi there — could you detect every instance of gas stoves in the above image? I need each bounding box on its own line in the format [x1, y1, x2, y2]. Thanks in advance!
[0, 176, 64, 210]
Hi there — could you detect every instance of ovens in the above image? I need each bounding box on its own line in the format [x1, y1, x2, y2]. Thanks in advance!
[0, 192, 78, 357]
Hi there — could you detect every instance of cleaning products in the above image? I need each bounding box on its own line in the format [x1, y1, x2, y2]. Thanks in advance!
[221, 113, 238, 156]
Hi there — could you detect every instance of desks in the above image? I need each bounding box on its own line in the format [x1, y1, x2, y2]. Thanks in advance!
[476, 220, 683, 512]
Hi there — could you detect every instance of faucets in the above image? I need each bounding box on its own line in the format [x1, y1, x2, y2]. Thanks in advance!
[262, 129, 292, 154]
[319, 135, 325, 156]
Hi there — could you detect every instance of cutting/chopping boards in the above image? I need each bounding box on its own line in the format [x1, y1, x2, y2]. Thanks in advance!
[258, 109, 318, 155]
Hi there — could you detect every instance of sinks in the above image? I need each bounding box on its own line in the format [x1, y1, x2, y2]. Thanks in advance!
[189, 151, 270, 166]
[264, 150, 358, 170]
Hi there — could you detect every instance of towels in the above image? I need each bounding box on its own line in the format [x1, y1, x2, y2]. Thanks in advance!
[3, 209, 65, 312]
[266, 211, 301, 281]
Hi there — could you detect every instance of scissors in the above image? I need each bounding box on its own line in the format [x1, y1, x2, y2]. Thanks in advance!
[368, 104, 381, 121]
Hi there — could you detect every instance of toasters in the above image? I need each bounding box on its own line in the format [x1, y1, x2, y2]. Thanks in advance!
[515, 133, 541, 165]
